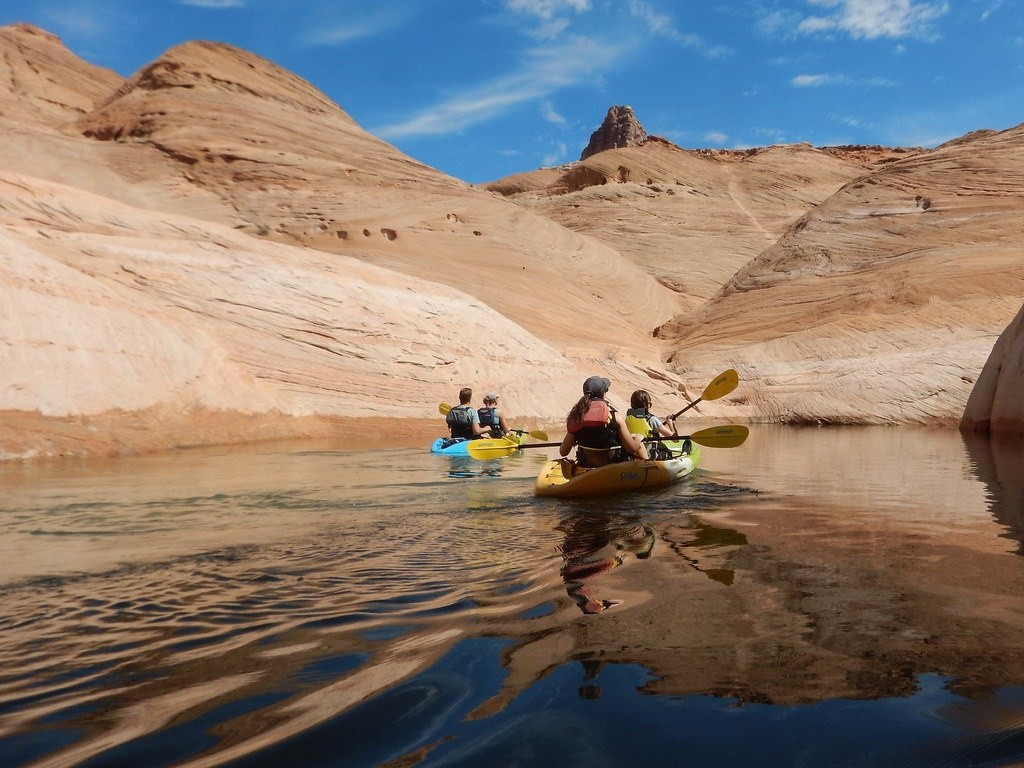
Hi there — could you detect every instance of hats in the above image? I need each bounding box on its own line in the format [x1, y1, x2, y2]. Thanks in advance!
[583, 376, 610, 397]
[486, 391, 499, 400]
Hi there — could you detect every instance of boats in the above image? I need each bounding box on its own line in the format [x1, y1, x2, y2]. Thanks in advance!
[431, 430, 526, 456]
[533, 438, 700, 498]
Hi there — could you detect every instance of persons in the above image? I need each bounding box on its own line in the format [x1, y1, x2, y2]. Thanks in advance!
[446, 387, 492, 438]
[477, 392, 512, 437]
[559, 375, 649, 467]
[625, 389, 675, 461]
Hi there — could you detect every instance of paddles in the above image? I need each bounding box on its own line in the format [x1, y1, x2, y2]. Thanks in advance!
[510, 427, 548, 441]
[438, 402, 520, 445]
[466, 425, 750, 460]
[661, 368, 739, 426]
